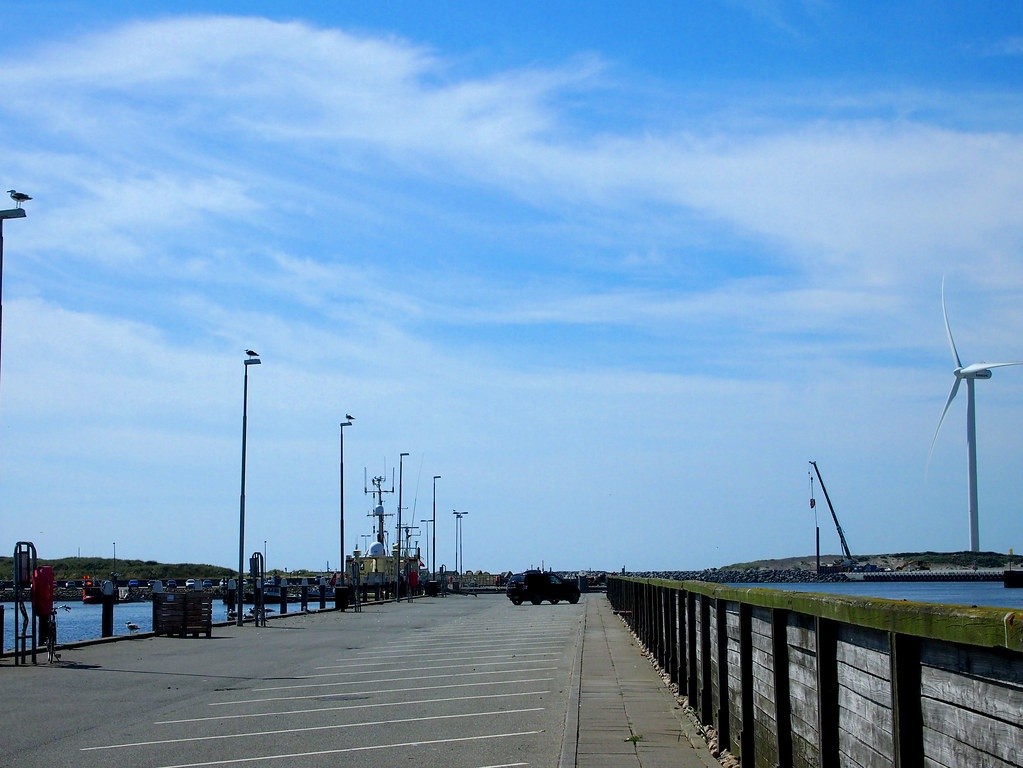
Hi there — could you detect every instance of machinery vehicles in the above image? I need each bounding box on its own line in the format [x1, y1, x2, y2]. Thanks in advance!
[809, 461, 863, 572]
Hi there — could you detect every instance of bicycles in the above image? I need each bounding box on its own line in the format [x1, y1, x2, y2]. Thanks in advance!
[46, 605, 72, 664]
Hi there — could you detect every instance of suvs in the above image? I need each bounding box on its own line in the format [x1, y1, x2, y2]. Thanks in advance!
[314, 575, 324, 585]
[505, 570, 581, 605]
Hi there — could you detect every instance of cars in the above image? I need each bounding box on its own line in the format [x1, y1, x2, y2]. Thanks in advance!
[147, 580, 157, 588]
[238, 578, 248, 586]
[165, 580, 177, 588]
[65, 581, 75, 588]
[202, 579, 213, 588]
[184, 578, 195, 588]
[128, 579, 139, 588]
[53, 581, 57, 587]
[219, 578, 228, 587]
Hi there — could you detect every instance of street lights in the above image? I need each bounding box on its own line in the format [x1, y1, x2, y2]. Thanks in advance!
[236, 358, 262, 626]
[453, 511, 467, 578]
[113, 542, 116, 582]
[432, 475, 442, 583]
[264, 541, 267, 578]
[421, 520, 433, 572]
[338, 421, 353, 611]
[396, 452, 410, 603]
